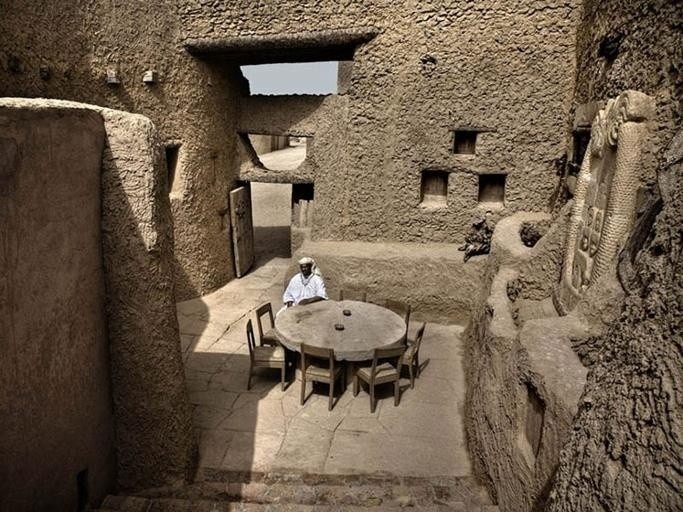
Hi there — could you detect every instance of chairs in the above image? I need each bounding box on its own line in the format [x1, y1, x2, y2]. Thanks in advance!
[299, 343, 345, 411]
[256, 302, 295, 369]
[351, 344, 406, 413]
[246, 319, 285, 391]
[402, 321, 427, 389]
[339, 289, 367, 302]
[386, 298, 411, 326]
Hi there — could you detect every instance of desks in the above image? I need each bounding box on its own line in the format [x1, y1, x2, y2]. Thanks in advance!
[274, 299, 408, 362]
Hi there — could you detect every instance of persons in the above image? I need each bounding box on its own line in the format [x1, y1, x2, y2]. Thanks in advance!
[282, 257, 328, 307]
